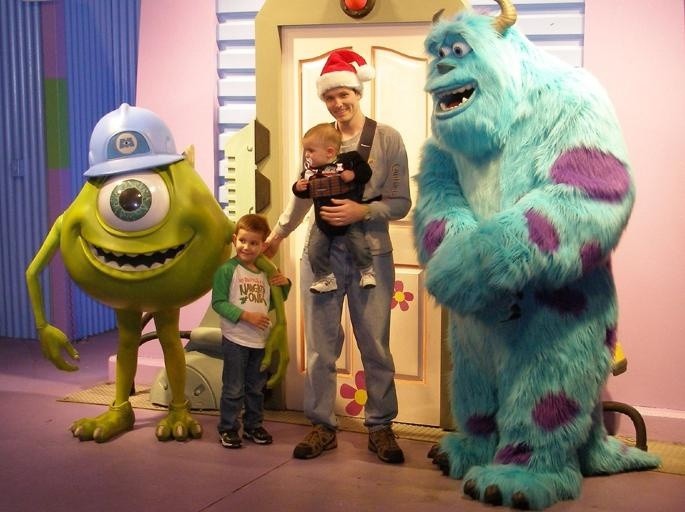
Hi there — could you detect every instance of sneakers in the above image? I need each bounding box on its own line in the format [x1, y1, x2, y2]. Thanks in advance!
[294, 424, 337, 458]
[309, 277, 338, 295]
[368, 427, 404, 463]
[360, 272, 376, 288]
[218, 430, 242, 448]
[242, 427, 272, 444]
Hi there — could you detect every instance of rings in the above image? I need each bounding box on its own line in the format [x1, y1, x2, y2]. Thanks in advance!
[336, 217, 341, 222]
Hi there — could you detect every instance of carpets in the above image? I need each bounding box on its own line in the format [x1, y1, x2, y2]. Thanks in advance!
[55, 378, 685, 475]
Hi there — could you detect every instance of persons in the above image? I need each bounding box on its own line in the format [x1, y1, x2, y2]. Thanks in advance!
[292, 123, 377, 294]
[262, 48, 412, 464]
[212, 213, 291, 450]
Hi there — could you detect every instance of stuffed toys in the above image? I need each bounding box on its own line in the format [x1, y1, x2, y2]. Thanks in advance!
[409, 0, 662, 512]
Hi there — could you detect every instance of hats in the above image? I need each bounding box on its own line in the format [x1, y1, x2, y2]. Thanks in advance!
[316, 48, 376, 100]
